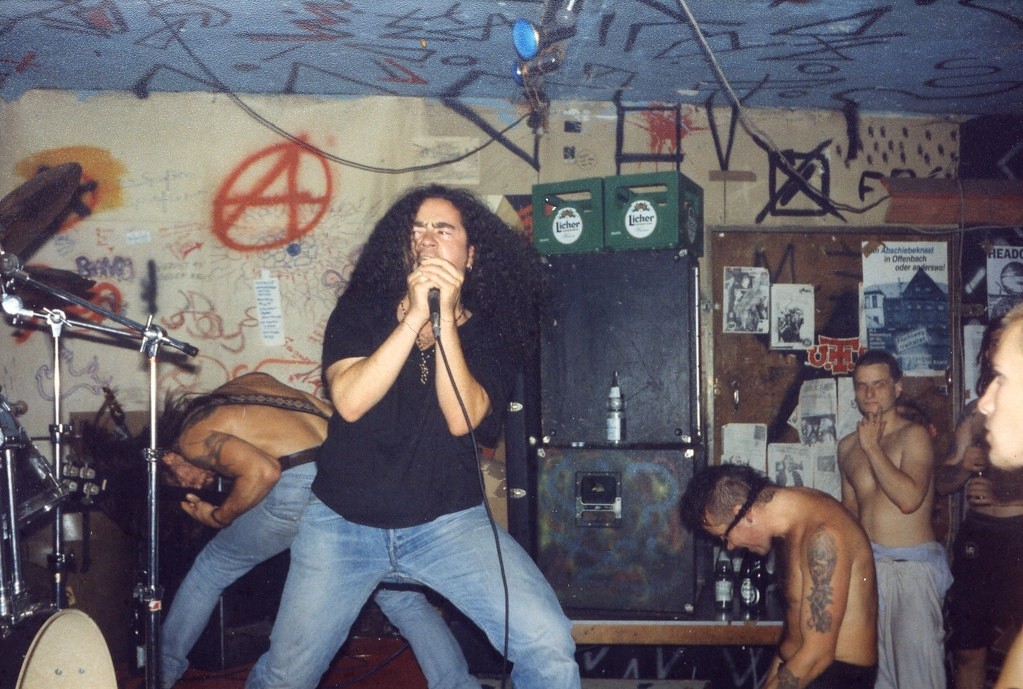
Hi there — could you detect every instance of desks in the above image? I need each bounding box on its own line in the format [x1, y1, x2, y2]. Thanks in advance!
[563, 571, 786, 645]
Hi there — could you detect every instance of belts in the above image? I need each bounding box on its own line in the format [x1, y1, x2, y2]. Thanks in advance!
[277, 445, 322, 473]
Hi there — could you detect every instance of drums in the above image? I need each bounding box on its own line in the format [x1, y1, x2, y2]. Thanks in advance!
[0, 387, 74, 542]
[0, 592, 119, 689]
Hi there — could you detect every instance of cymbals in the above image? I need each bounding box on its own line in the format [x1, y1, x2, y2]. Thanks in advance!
[0, 163, 84, 260]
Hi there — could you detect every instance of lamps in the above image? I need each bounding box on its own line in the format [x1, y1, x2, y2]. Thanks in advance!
[511, 9, 578, 61]
[510, 52, 560, 88]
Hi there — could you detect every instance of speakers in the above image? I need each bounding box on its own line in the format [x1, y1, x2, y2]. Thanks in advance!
[537, 447, 708, 612]
[158, 487, 292, 673]
[540, 249, 703, 447]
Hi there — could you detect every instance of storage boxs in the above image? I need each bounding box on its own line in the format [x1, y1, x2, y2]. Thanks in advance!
[532, 177, 605, 253]
[605, 170, 704, 260]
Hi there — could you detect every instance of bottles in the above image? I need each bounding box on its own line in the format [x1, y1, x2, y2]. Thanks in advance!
[740, 552, 754, 620]
[132, 580, 144, 606]
[974, 440, 990, 505]
[753, 557, 767, 620]
[606, 371, 626, 443]
[130, 610, 148, 676]
[715, 546, 734, 613]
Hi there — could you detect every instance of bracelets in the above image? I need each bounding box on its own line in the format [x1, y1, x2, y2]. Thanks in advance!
[212, 508, 232, 525]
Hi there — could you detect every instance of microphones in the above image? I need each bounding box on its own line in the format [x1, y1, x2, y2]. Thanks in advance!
[428, 286, 440, 338]
[103, 386, 125, 427]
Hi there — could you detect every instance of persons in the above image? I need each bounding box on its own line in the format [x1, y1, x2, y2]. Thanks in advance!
[132, 371, 482, 689]
[243, 185, 581, 689]
[777, 456, 803, 487]
[977, 300, 1023, 689]
[935, 317, 1023, 689]
[837, 348, 955, 688]
[805, 418, 835, 443]
[680, 463, 878, 689]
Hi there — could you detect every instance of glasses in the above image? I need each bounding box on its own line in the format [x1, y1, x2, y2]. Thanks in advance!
[714, 508, 744, 551]
